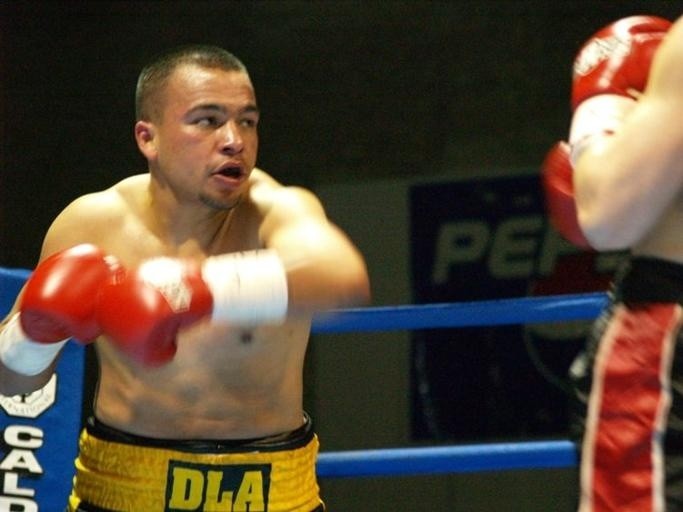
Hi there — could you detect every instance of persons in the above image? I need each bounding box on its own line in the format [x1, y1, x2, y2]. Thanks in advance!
[0, 42, 373, 512]
[541, 14, 683, 512]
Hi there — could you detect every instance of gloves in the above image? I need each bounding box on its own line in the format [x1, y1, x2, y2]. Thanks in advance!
[0, 244, 124, 377]
[540, 142, 591, 254]
[568, 13, 675, 155]
[98, 246, 289, 367]
